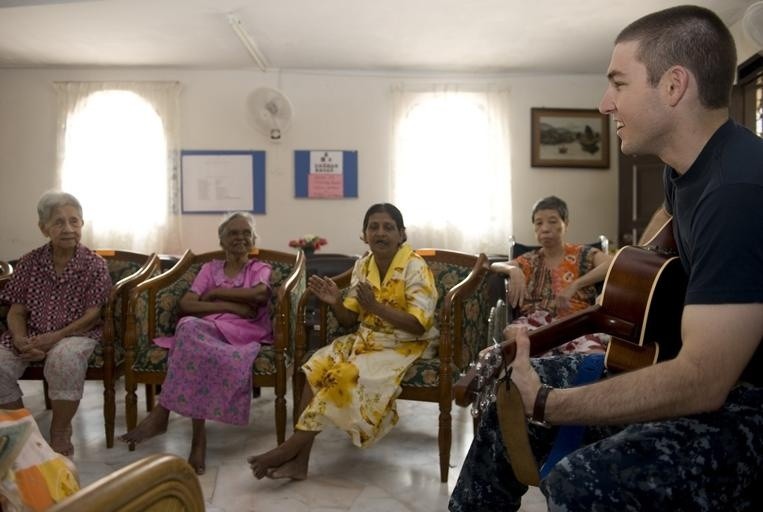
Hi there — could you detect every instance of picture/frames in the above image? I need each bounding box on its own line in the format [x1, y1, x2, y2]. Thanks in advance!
[529, 108, 610, 170]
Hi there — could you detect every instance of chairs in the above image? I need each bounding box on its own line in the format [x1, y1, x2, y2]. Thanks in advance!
[123, 246, 305, 454]
[0, 246, 157, 448]
[290, 246, 489, 485]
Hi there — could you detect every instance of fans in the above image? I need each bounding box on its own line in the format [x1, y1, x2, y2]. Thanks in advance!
[246, 86, 295, 142]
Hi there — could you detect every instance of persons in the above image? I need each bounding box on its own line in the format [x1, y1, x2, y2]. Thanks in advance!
[487, 194, 614, 356]
[243, 202, 438, 482]
[445, 5, 763, 511]
[0, 189, 112, 458]
[115, 210, 275, 476]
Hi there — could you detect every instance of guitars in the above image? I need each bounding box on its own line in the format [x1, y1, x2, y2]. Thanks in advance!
[453, 245, 688, 418]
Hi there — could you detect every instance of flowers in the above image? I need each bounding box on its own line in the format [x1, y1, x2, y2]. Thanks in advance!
[288, 234, 327, 257]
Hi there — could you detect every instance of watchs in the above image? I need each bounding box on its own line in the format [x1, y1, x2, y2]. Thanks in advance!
[526, 382, 552, 431]
